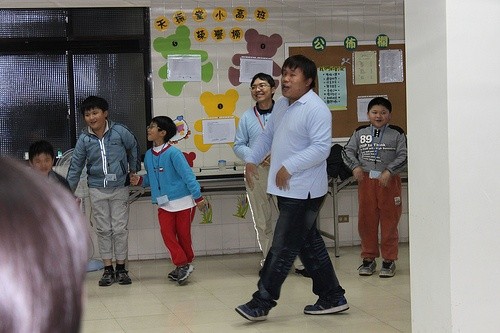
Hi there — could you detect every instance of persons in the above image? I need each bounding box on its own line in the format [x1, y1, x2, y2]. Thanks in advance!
[235, 55, 349, 321]
[129, 116, 207, 284]
[342, 97, 408, 277]
[233, 73, 311, 277]
[0, 153, 91, 333]
[24, 141, 74, 197]
[66, 95, 141, 286]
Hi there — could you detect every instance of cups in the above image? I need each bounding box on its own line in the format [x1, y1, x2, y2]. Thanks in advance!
[218, 160, 226, 172]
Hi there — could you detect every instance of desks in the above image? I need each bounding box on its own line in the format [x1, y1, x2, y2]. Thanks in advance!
[317, 171, 408, 258]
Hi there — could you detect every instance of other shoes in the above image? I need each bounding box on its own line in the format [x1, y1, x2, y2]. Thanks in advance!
[380, 260, 396, 277]
[115, 268, 132, 284]
[99, 268, 115, 286]
[295, 267, 310, 278]
[356, 258, 377, 275]
[168, 268, 177, 281]
[177, 264, 195, 284]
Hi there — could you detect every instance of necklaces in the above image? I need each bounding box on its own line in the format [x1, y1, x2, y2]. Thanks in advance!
[254, 106, 265, 130]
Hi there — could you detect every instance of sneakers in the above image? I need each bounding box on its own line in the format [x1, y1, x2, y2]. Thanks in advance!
[234, 298, 269, 321]
[304, 293, 349, 314]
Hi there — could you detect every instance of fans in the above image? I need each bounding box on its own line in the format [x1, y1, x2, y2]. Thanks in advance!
[55, 147, 106, 273]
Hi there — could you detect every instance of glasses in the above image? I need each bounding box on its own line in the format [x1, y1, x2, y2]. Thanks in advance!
[147, 125, 160, 130]
[249, 85, 271, 91]
